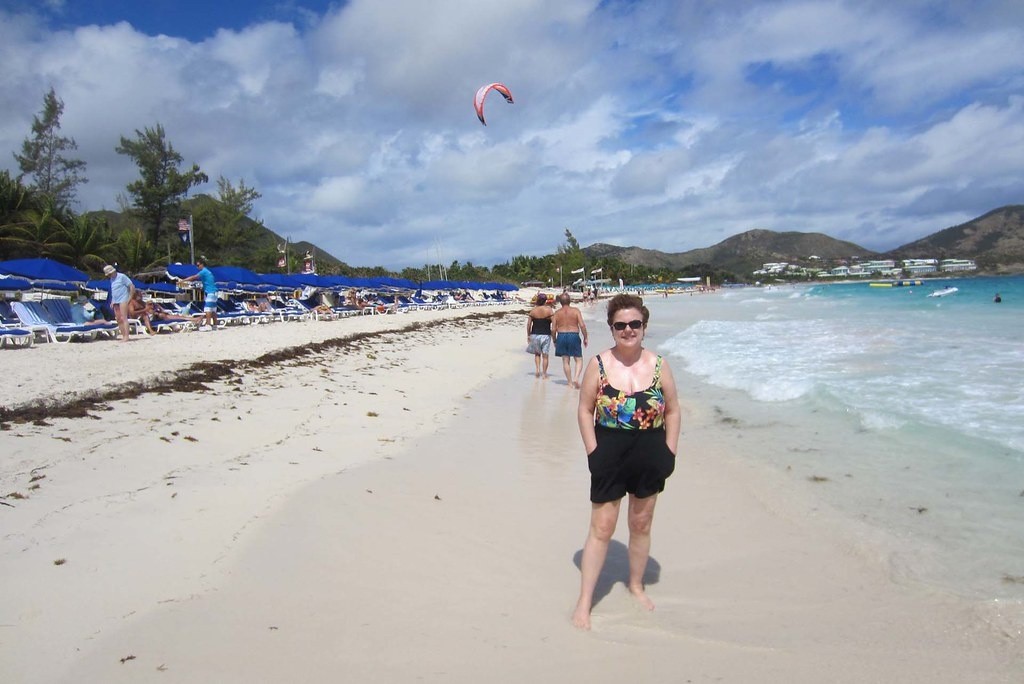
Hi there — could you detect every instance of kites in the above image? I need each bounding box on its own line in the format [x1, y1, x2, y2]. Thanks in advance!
[474, 82, 515, 127]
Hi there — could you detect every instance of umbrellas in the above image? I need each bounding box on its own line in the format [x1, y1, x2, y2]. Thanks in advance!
[1, 255, 519, 302]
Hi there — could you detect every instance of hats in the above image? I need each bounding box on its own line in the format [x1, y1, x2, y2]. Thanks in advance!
[538, 294, 547, 300]
[77, 295, 88, 303]
[103, 265, 117, 276]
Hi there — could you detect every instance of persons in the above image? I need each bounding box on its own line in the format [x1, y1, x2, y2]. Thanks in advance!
[176, 258, 218, 332]
[70, 295, 112, 326]
[127, 290, 159, 337]
[146, 300, 204, 324]
[527, 293, 555, 379]
[571, 292, 681, 631]
[246, 301, 272, 313]
[103, 265, 134, 343]
[530, 284, 715, 306]
[995, 293, 1001, 303]
[311, 303, 398, 321]
[551, 292, 588, 389]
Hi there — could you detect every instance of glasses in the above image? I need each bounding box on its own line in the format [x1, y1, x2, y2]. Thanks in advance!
[197, 264, 201, 267]
[611, 320, 645, 330]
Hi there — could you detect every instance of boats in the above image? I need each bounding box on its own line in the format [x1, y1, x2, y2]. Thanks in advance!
[928, 286, 958, 296]
[654, 286, 698, 294]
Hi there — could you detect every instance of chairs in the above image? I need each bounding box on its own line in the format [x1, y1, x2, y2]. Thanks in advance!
[0, 292, 509, 348]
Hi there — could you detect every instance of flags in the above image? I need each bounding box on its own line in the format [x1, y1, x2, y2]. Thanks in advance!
[178, 218, 190, 230]
[180, 231, 190, 245]
